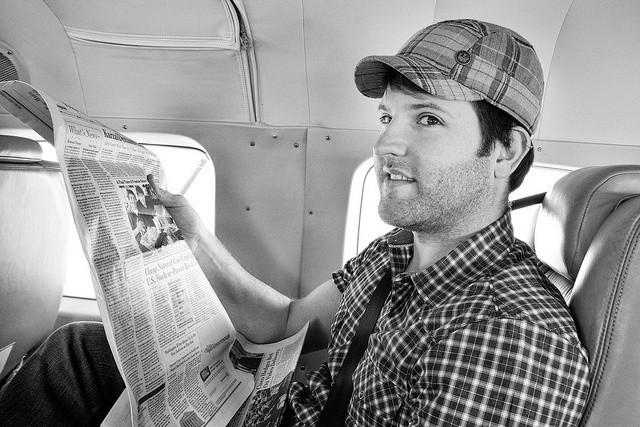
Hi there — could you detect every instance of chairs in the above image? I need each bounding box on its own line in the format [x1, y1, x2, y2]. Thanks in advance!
[531, 165, 640, 427]
[0, 135, 70, 393]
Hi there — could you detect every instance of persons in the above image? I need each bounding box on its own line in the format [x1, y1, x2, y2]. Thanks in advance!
[126, 190, 153, 251]
[1, 18, 591, 426]
[229, 351, 262, 377]
[136, 186, 158, 227]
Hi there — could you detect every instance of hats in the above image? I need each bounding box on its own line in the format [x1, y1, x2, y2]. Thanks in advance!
[352, 18, 545, 135]
